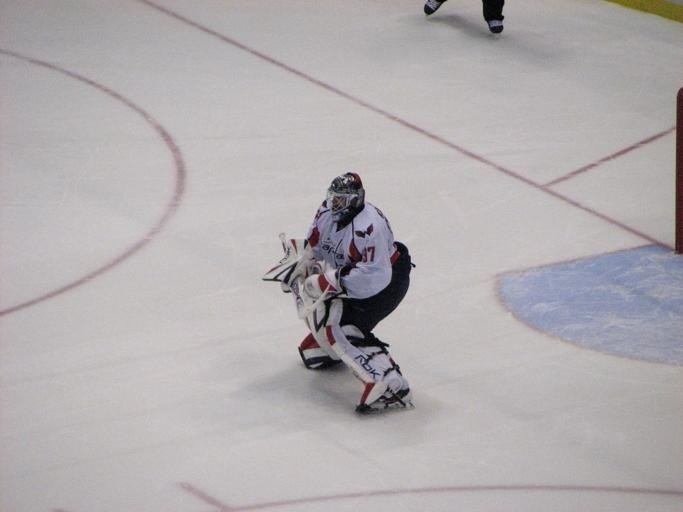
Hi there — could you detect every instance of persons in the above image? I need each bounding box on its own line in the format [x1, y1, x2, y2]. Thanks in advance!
[261, 171, 413, 411]
[424, 0, 506, 34]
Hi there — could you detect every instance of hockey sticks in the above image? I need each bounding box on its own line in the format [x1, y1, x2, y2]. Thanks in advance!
[279, 233, 331, 319]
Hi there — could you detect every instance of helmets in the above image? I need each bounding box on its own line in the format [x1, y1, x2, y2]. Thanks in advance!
[326, 171, 365, 217]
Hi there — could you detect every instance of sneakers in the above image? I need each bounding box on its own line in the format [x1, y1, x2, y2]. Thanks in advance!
[424, 0, 447, 15]
[375, 375, 411, 403]
[487, 16, 504, 34]
